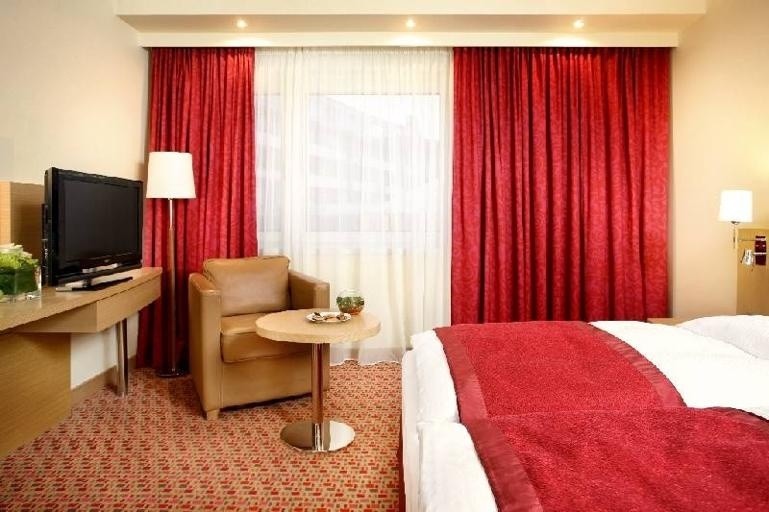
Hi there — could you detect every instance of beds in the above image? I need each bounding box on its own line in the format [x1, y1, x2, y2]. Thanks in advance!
[397, 226, 768, 512]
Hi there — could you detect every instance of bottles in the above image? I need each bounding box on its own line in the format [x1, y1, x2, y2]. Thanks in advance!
[336, 288, 365, 316]
[755, 235, 768, 267]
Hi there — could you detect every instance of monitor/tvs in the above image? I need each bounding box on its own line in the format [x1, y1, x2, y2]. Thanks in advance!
[42, 167, 143, 292]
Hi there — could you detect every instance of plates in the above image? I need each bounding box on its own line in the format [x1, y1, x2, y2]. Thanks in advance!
[305, 310, 352, 324]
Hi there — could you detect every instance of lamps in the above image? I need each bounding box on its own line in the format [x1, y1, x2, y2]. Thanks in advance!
[146, 151, 198, 378]
[718, 189, 767, 267]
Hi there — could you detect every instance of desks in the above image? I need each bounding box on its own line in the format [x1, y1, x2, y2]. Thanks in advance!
[255, 307, 380, 454]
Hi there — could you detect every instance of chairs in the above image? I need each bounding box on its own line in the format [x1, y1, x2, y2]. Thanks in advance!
[188, 253, 331, 423]
